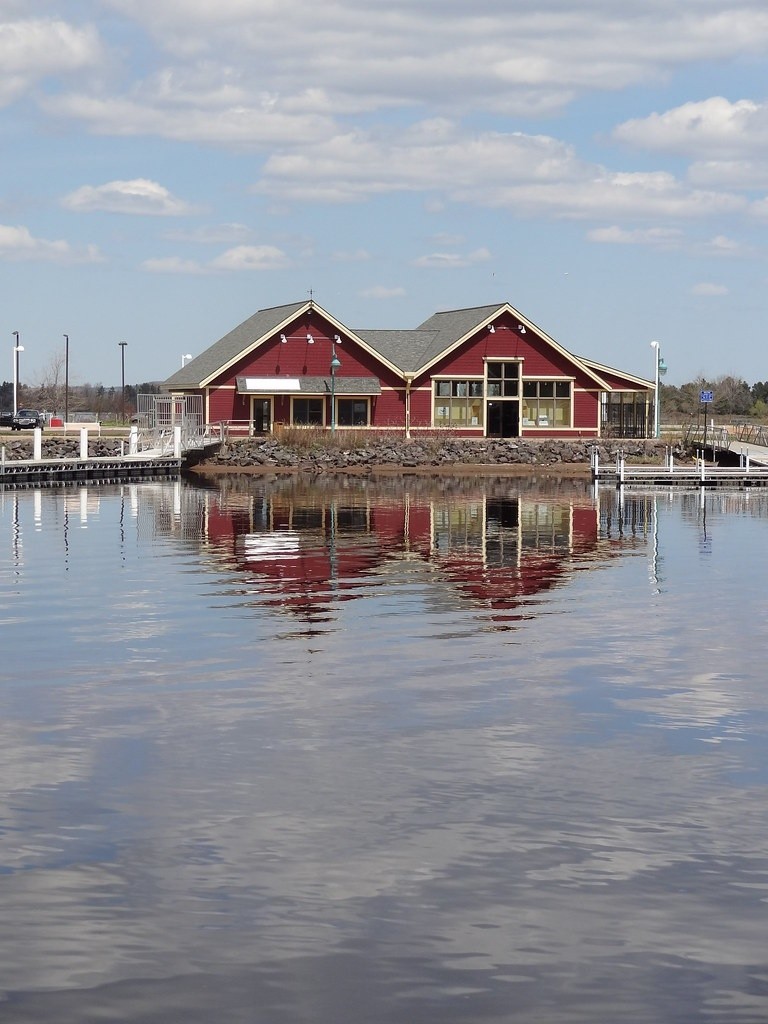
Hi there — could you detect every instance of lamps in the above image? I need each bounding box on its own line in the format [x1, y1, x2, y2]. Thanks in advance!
[518, 324, 526, 334]
[334, 335, 342, 344]
[487, 324, 496, 333]
[280, 334, 288, 343]
[307, 334, 314, 344]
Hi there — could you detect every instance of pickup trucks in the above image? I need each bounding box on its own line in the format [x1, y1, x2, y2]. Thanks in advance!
[11, 410, 44, 430]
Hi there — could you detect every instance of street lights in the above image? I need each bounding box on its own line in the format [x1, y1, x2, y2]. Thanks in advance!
[658, 357, 667, 439]
[331, 359, 342, 437]
[650, 341, 659, 439]
[119, 341, 128, 425]
[12, 346, 24, 416]
[64, 334, 68, 422]
[12, 330, 19, 410]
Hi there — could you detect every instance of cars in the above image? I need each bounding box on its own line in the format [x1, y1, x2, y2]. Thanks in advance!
[132, 409, 154, 426]
[0, 412, 13, 427]
